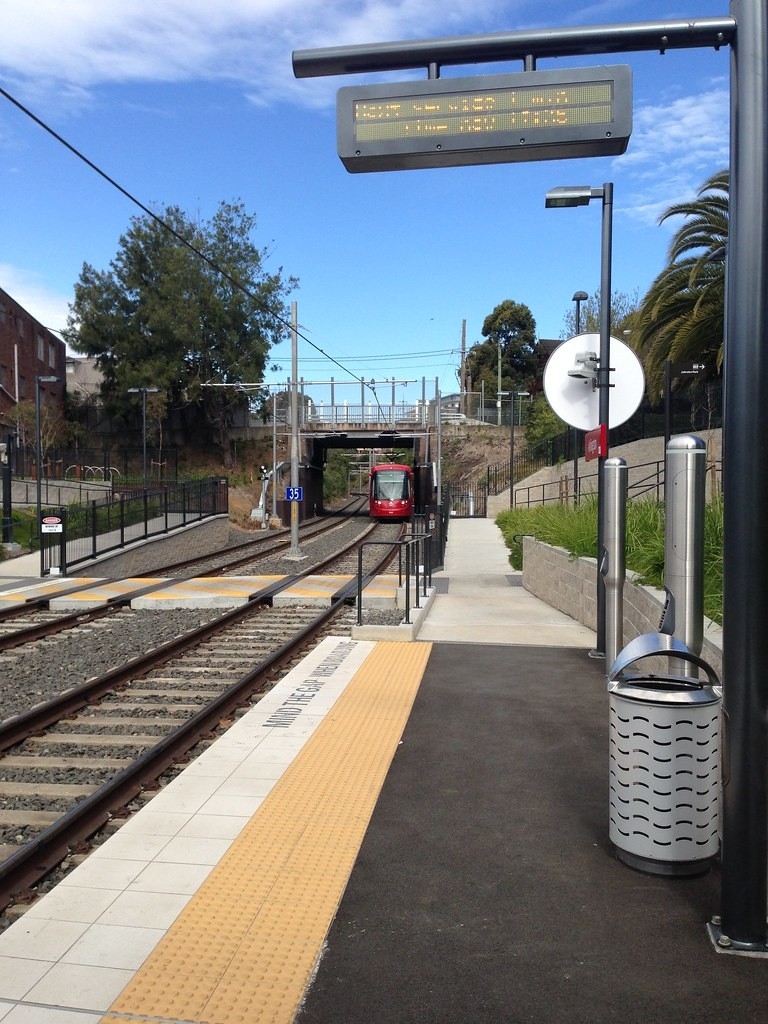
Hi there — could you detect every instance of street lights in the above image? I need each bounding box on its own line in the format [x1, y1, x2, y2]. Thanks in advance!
[572, 292, 589, 509]
[35, 376, 61, 538]
[127, 387, 161, 488]
[544, 184, 615, 659]
[496, 391, 530, 511]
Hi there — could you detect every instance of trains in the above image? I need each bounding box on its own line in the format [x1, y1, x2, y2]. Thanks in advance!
[368, 465, 416, 517]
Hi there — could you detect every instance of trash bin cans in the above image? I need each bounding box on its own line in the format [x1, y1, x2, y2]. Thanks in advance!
[605, 633, 722, 875]
